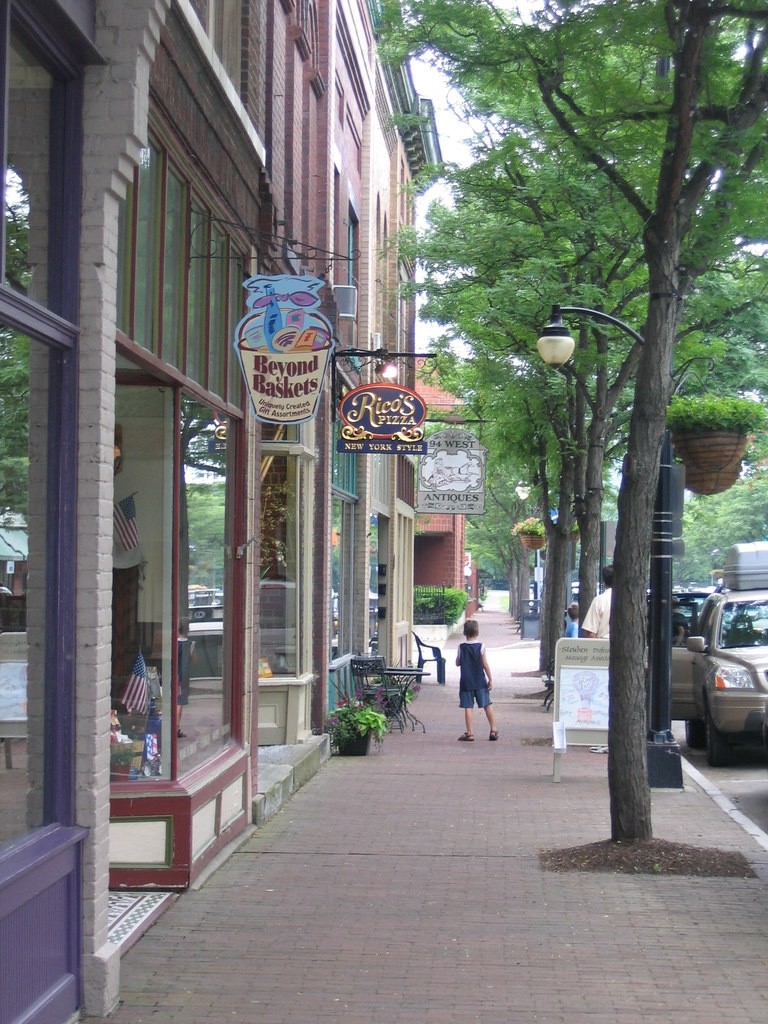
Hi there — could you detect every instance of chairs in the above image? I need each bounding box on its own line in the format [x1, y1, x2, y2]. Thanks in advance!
[348, 653, 426, 735]
[411, 631, 446, 685]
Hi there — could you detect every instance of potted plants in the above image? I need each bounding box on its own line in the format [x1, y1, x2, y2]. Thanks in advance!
[665, 388, 768, 495]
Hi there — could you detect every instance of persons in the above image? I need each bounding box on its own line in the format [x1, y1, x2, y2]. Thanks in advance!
[672, 596, 689, 646]
[177, 617, 197, 737]
[565, 602, 579, 637]
[456, 620, 499, 741]
[581, 564, 612, 638]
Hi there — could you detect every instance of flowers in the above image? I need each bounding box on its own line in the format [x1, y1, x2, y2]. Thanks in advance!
[508, 516, 548, 535]
[320, 671, 390, 753]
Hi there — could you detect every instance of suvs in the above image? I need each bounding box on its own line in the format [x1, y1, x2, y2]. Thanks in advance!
[668, 542, 768, 769]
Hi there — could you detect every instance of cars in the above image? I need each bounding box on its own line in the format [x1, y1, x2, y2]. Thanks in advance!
[570, 580, 719, 631]
[188, 580, 339, 674]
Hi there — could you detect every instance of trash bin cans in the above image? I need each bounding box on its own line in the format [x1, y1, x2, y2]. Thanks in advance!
[520, 599, 541, 640]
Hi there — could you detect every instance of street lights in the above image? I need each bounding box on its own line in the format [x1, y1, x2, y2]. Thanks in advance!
[534, 304, 684, 789]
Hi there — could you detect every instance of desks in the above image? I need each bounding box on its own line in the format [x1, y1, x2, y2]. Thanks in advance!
[375, 671, 432, 731]
[384, 665, 423, 671]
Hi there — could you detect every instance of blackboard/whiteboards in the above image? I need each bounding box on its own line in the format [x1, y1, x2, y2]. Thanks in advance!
[559, 664, 609, 733]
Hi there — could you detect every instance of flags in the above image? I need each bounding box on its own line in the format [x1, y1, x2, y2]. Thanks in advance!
[119, 651, 148, 715]
[113, 495, 140, 551]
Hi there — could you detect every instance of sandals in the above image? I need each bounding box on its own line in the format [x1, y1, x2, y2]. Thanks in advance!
[490, 731, 498, 740]
[458, 734, 474, 741]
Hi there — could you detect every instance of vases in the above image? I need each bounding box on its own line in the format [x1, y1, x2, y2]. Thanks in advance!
[336, 726, 373, 756]
